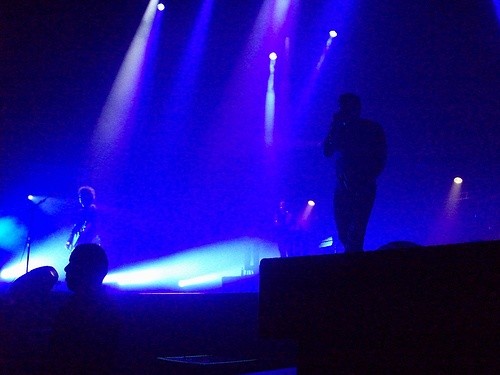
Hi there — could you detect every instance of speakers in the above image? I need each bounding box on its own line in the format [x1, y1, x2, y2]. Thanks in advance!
[258, 236, 498, 374]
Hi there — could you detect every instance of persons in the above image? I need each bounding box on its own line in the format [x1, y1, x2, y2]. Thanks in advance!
[320, 94, 384, 250]
[51, 244, 117, 375]
[68, 186, 101, 244]
